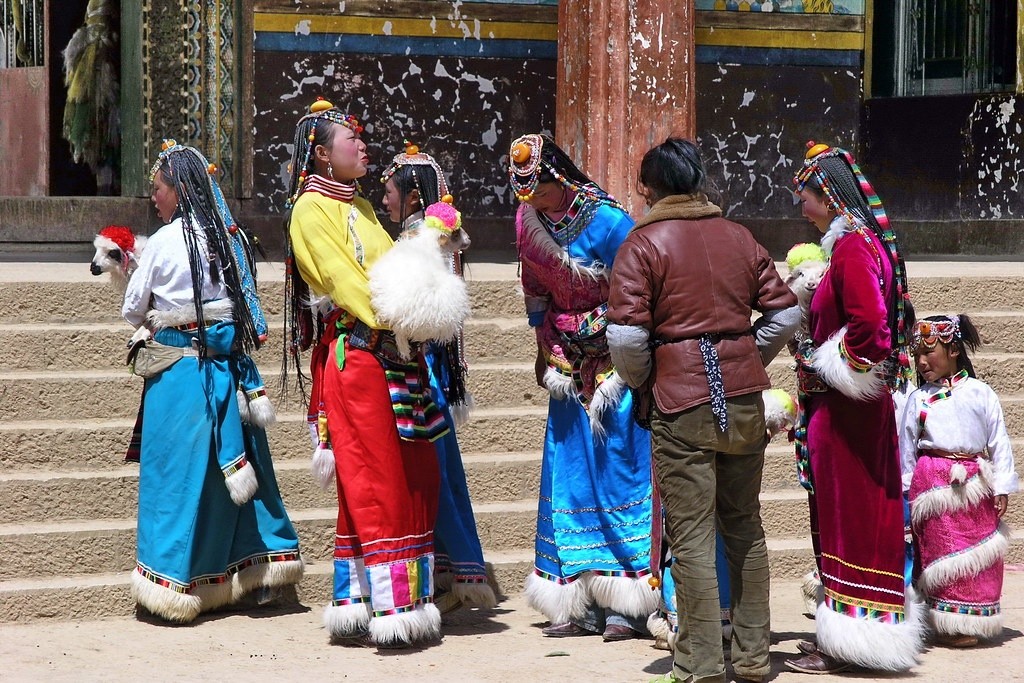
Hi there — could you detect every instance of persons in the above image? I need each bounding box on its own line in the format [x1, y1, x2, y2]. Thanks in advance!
[779, 140, 919, 675]
[121, 139, 304, 626]
[898, 314, 1014, 647]
[606, 134, 801, 683]
[506, 131, 665, 640]
[379, 139, 495, 617]
[281, 97, 441, 648]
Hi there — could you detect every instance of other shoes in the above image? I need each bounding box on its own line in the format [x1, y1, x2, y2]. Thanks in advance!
[948, 635, 978, 647]
[542, 622, 590, 636]
[602, 625, 636, 641]
[784, 642, 852, 673]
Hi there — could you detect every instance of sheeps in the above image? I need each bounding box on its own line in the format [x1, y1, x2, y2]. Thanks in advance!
[90, 226, 148, 296]
[784, 260, 827, 301]
[367, 202, 473, 362]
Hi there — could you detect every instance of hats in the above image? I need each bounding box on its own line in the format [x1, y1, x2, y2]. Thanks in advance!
[105, 227, 134, 254]
